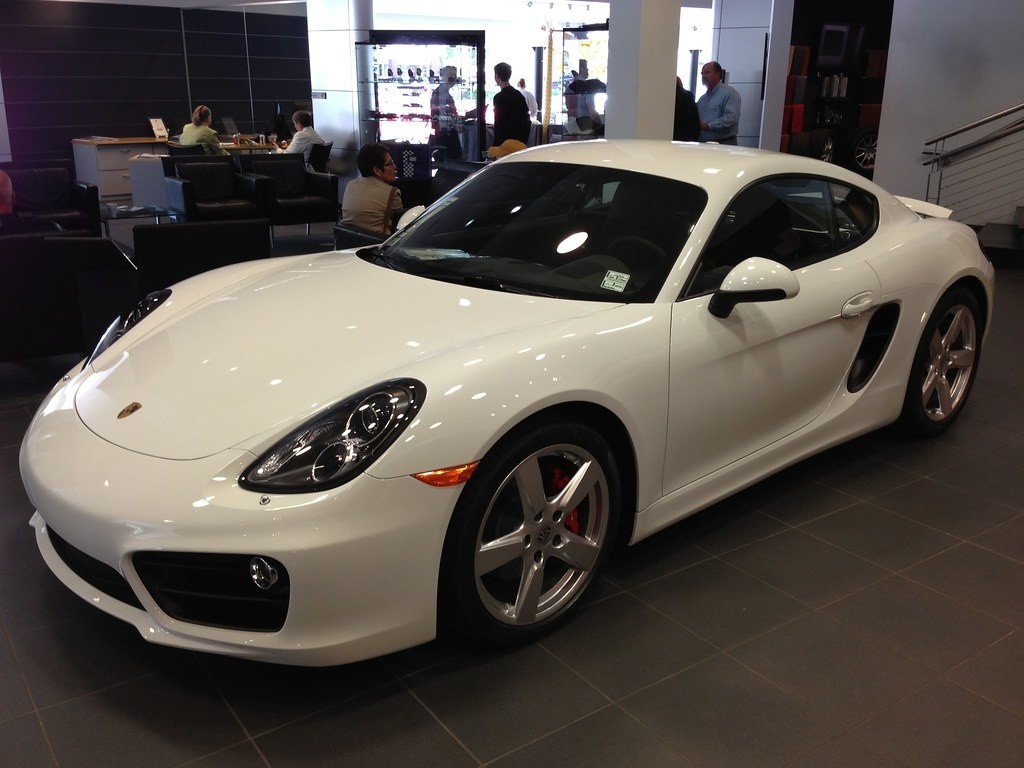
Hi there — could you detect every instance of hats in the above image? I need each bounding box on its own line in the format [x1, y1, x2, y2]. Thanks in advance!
[489, 139, 528, 157]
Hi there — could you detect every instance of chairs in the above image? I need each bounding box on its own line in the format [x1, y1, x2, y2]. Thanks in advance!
[0, 141, 444, 365]
[706, 186, 793, 268]
[589, 177, 670, 256]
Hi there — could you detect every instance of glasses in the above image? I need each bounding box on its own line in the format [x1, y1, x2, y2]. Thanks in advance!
[384, 159, 393, 166]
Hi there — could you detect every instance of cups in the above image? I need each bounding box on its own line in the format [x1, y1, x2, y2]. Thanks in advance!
[106, 203, 118, 218]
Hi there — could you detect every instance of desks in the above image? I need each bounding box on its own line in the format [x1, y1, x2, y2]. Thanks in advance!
[98, 200, 185, 241]
[462, 119, 553, 163]
[219, 141, 292, 154]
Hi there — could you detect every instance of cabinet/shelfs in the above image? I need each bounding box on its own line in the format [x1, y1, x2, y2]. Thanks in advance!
[561, 23, 610, 142]
[356, 28, 485, 207]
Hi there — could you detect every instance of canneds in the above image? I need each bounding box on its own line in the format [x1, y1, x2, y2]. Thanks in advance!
[233, 133, 241, 146]
[260, 134, 265, 146]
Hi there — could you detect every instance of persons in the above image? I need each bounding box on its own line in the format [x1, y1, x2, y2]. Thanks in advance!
[492, 62, 531, 146]
[179, 106, 230, 156]
[490, 140, 528, 160]
[673, 76, 701, 142]
[431, 66, 468, 160]
[272, 111, 325, 173]
[565, 59, 601, 134]
[0, 171, 63, 236]
[517, 79, 537, 120]
[696, 61, 741, 146]
[342, 143, 404, 235]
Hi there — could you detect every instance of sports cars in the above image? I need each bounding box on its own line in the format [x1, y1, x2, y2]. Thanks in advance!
[19, 141, 996, 670]
